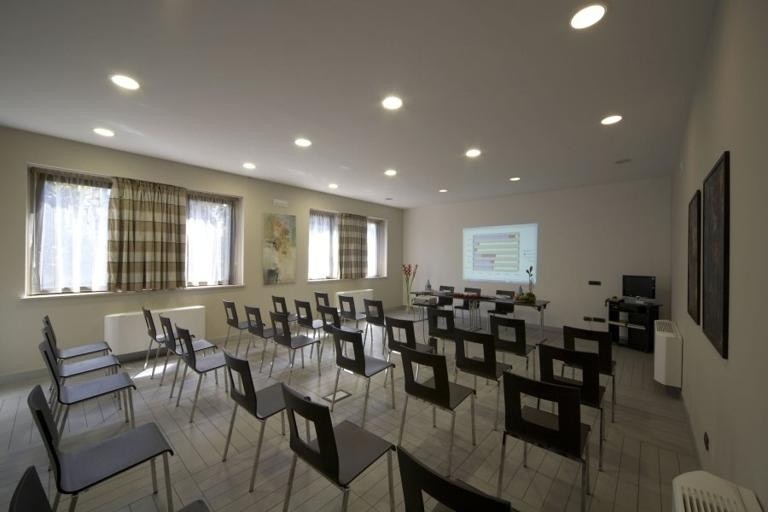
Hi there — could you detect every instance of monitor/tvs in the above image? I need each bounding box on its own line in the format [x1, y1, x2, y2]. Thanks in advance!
[623, 275, 656, 299]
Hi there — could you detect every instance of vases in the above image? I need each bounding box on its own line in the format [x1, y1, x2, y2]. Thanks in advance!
[525, 263, 536, 307]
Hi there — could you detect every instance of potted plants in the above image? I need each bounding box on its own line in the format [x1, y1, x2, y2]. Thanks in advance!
[336, 288, 375, 321]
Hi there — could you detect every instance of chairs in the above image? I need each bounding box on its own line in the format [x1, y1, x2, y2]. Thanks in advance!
[454, 327, 512, 389]
[427, 308, 455, 355]
[271, 295, 306, 336]
[223, 301, 266, 359]
[330, 323, 396, 431]
[159, 313, 219, 398]
[497, 368, 591, 512]
[313, 291, 341, 321]
[490, 315, 535, 370]
[290, 299, 338, 365]
[43, 313, 113, 401]
[42, 326, 122, 418]
[535, 342, 607, 451]
[173, 320, 243, 424]
[437, 284, 454, 325]
[281, 383, 398, 511]
[452, 286, 481, 329]
[268, 310, 321, 385]
[27, 383, 175, 512]
[561, 325, 616, 424]
[222, 349, 313, 494]
[140, 304, 197, 379]
[364, 297, 391, 356]
[338, 294, 373, 338]
[0, 463, 211, 511]
[396, 443, 513, 512]
[398, 343, 478, 478]
[245, 305, 283, 373]
[39, 340, 139, 439]
[486, 288, 515, 330]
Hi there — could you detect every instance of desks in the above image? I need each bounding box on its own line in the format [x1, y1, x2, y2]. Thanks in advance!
[605, 294, 662, 352]
[409, 290, 551, 341]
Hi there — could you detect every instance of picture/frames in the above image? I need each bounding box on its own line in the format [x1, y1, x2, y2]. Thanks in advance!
[687, 186, 700, 328]
[702, 148, 731, 359]
